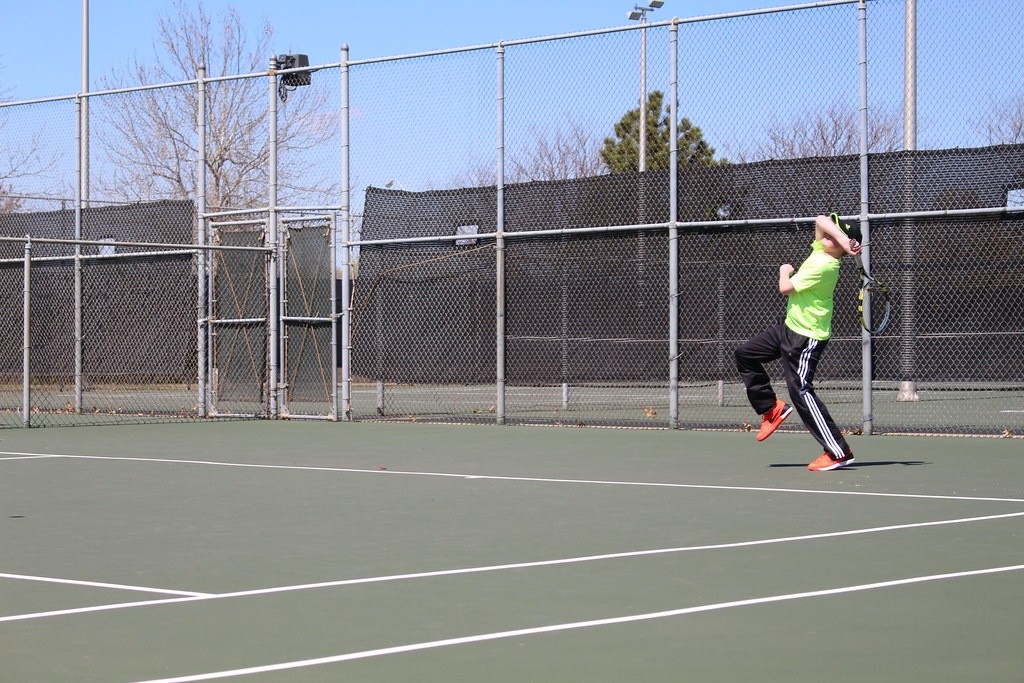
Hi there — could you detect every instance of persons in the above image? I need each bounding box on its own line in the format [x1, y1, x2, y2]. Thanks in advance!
[733, 212, 864, 472]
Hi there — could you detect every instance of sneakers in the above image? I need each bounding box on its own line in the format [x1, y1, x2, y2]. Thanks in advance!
[806, 452, 856, 471]
[755, 399, 794, 441]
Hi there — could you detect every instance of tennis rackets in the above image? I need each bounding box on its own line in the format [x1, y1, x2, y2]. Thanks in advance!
[849, 238, 891, 334]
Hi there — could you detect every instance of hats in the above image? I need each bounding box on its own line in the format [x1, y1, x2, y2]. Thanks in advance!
[831, 213, 862, 244]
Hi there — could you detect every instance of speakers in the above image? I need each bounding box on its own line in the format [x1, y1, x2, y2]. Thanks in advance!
[282, 54, 311, 86]
